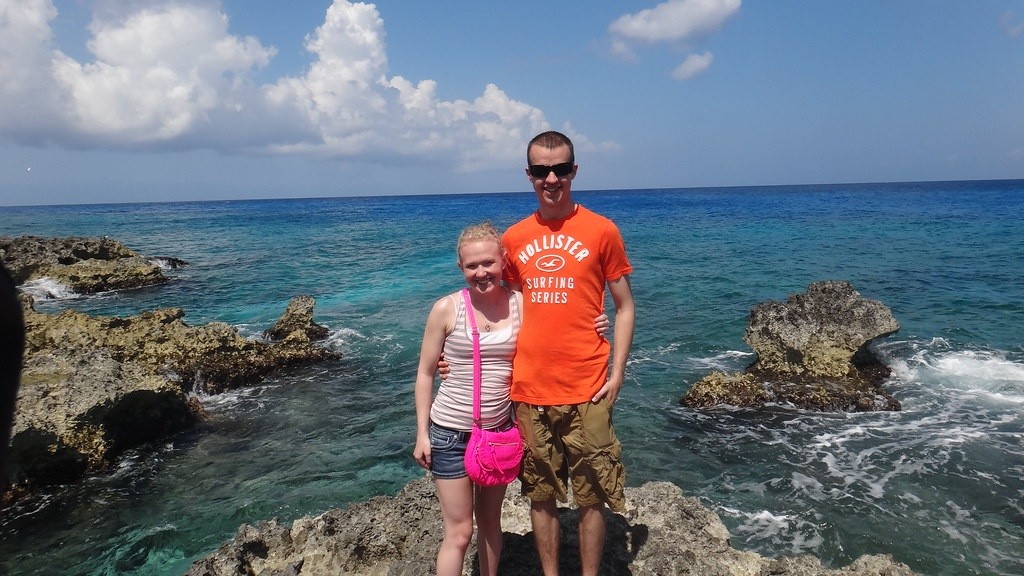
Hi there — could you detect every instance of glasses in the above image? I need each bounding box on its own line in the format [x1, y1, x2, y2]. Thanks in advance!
[527, 161, 575, 178]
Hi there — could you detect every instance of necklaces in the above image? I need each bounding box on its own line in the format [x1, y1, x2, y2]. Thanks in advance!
[481, 288, 501, 331]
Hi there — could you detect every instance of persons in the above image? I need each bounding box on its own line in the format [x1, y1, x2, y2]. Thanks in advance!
[436, 130, 635, 576]
[413, 221, 610, 575]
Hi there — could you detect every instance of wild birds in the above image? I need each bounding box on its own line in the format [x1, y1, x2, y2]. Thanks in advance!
[24, 167, 32, 175]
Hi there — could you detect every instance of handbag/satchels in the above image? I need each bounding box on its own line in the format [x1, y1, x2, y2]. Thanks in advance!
[464, 424, 526, 487]
